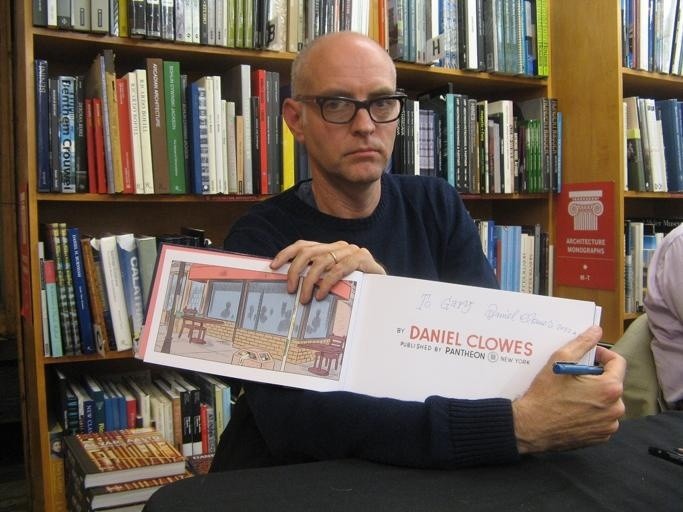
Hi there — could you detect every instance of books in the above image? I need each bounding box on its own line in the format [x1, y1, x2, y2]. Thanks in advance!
[33, 49, 311, 195]
[32, 1, 550, 78]
[39, 222, 212, 357]
[46, 362, 246, 512]
[134, 242, 601, 403]
[386, 87, 562, 193]
[622, 0, 682, 312]
[474, 219, 554, 297]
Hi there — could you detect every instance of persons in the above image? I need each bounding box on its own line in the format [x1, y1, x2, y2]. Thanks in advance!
[643, 223, 681, 411]
[210, 34, 628, 473]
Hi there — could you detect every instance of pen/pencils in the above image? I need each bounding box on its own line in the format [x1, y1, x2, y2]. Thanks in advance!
[554, 362, 603, 375]
[676, 448, 683, 453]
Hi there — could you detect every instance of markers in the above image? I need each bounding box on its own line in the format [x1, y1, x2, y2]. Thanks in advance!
[649, 446, 683, 465]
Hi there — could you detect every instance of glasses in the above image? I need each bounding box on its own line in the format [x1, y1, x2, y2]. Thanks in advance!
[295, 88, 408, 123]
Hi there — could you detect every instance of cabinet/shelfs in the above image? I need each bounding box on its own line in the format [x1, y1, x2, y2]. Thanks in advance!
[553, 2, 683, 352]
[5, 0, 553, 510]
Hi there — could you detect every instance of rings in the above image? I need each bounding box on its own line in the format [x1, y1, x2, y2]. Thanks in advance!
[329, 251, 337, 264]
